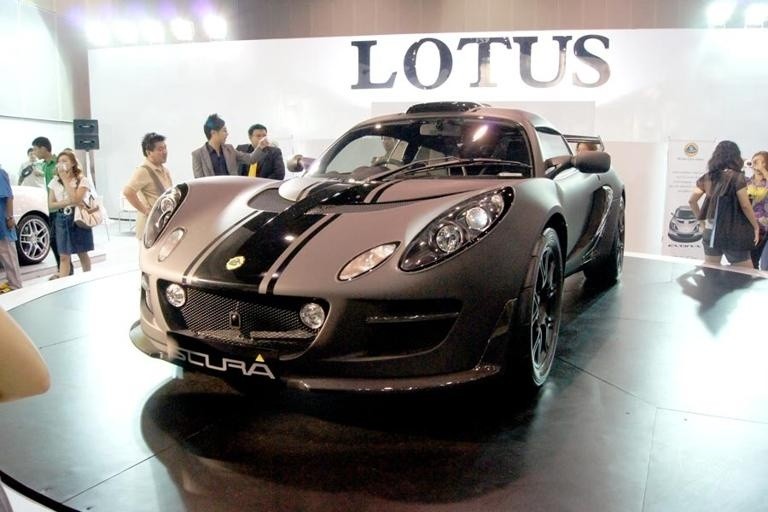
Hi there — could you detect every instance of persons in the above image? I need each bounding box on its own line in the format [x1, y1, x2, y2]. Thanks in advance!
[370, 134, 398, 166]
[191, 113, 270, 177]
[16, 137, 95, 279]
[688, 139, 760, 270]
[0, 169, 23, 293]
[740, 151, 768, 271]
[234, 123, 287, 182]
[0, 308, 52, 405]
[575, 141, 598, 155]
[124, 131, 173, 241]
[675, 265, 768, 335]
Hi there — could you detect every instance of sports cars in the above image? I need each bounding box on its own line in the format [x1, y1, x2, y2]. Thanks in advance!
[126, 97, 630, 404]
[666, 204, 705, 244]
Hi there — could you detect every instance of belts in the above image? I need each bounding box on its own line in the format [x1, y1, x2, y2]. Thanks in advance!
[58, 206, 74, 215]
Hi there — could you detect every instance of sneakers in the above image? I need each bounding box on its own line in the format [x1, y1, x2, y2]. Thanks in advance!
[0, 282, 11, 293]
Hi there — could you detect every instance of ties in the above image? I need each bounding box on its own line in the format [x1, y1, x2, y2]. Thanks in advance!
[248, 161, 257, 176]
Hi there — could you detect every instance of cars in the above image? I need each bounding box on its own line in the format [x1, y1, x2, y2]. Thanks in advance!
[1, 182, 58, 268]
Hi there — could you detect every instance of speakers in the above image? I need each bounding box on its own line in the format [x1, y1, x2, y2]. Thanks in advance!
[72, 118, 100, 150]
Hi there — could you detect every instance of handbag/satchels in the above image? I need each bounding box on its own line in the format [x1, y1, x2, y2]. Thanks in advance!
[73, 199, 104, 229]
[714, 195, 767, 250]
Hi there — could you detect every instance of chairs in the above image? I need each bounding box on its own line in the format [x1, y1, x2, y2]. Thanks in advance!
[412, 136, 530, 176]
